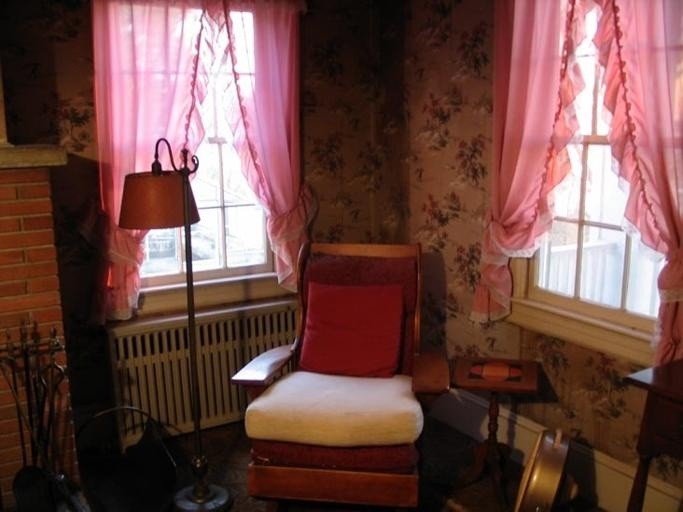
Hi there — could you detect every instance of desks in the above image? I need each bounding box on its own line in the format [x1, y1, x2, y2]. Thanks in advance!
[624, 358, 683, 512]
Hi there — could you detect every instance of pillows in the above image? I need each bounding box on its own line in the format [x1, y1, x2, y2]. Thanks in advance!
[297, 282, 405, 378]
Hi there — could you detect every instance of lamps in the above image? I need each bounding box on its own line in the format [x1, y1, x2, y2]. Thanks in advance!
[118, 137, 229, 511]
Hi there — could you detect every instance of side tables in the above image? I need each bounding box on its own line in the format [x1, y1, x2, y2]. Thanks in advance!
[452, 356, 542, 511]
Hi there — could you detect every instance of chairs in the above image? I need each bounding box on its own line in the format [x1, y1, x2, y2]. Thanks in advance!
[232, 242, 451, 512]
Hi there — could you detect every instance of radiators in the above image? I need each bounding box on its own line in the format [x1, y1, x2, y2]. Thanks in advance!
[106, 301, 299, 454]
